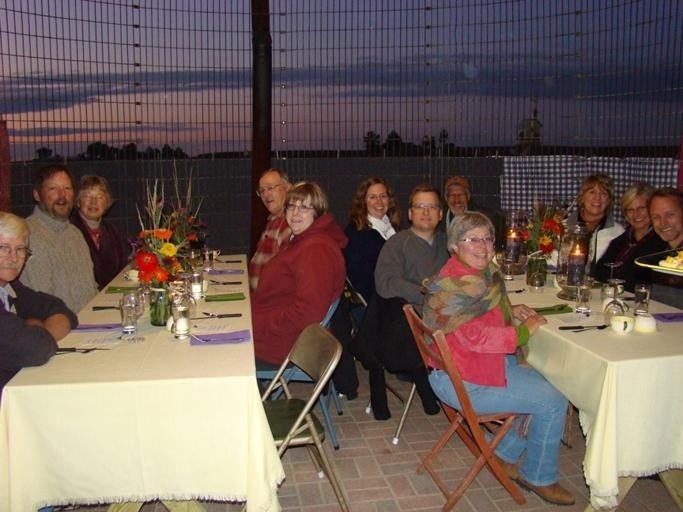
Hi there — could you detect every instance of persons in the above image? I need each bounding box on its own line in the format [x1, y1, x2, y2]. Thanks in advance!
[19, 165, 100, 315]
[67, 175, 130, 292]
[443, 175, 479, 235]
[591, 182, 666, 294]
[251, 183, 348, 371]
[348, 177, 441, 421]
[569, 174, 625, 276]
[607, 188, 682, 309]
[418, 213, 576, 505]
[249, 167, 304, 292]
[374, 181, 450, 320]
[0, 211, 78, 406]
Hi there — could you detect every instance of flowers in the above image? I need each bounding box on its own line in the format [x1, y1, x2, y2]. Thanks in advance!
[515, 194, 580, 259]
[134, 159, 203, 284]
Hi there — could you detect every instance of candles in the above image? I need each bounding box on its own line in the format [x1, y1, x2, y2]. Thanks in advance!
[571, 244, 583, 255]
[508, 230, 517, 237]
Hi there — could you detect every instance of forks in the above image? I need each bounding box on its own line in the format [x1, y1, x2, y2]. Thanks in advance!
[536, 304, 567, 313]
[573, 324, 606, 333]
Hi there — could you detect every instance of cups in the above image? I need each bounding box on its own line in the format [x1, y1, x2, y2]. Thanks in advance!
[174, 303, 190, 340]
[211, 249, 221, 259]
[123, 271, 145, 318]
[202, 280, 208, 293]
[172, 273, 197, 327]
[610, 315, 635, 335]
[502, 258, 513, 281]
[604, 284, 624, 296]
[575, 280, 592, 314]
[528, 257, 546, 295]
[200, 246, 213, 267]
[633, 281, 652, 316]
[186, 248, 203, 298]
[117, 295, 137, 334]
[634, 312, 657, 334]
[602, 278, 626, 326]
[600, 260, 625, 304]
[603, 296, 630, 313]
[123, 269, 140, 282]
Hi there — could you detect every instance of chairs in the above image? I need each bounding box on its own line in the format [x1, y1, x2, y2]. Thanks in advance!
[346, 274, 416, 445]
[256, 295, 344, 450]
[403, 304, 526, 512]
[262, 321, 350, 508]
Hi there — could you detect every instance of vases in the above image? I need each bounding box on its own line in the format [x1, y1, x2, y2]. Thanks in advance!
[526, 258, 549, 286]
[147, 282, 173, 328]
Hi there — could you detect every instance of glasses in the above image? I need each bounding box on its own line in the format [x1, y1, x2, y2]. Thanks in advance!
[287, 200, 315, 213]
[0, 246, 33, 259]
[411, 202, 441, 211]
[458, 237, 496, 244]
[256, 182, 286, 196]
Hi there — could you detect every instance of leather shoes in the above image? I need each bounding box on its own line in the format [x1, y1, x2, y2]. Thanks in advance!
[517, 477, 575, 506]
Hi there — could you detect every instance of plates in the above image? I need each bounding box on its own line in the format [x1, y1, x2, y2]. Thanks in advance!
[634, 246, 683, 277]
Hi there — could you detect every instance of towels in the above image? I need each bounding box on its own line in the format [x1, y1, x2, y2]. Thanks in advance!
[209, 269, 244, 275]
[205, 293, 245, 302]
[533, 305, 574, 315]
[190, 330, 250, 344]
[105, 286, 137, 294]
[652, 313, 683, 324]
[69, 323, 124, 332]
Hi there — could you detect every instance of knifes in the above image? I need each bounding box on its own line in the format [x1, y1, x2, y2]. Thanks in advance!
[91, 305, 120, 311]
[558, 325, 599, 331]
[56, 348, 111, 352]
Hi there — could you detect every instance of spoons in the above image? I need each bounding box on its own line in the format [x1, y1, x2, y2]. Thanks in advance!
[189, 332, 244, 343]
[216, 259, 242, 264]
[210, 279, 242, 286]
[201, 310, 242, 318]
